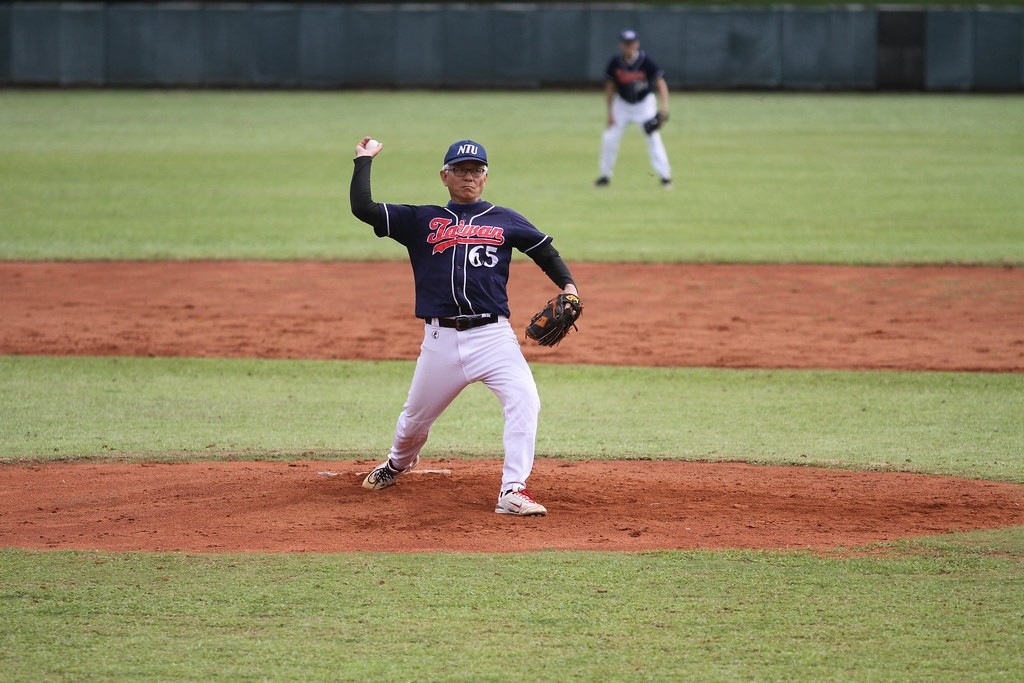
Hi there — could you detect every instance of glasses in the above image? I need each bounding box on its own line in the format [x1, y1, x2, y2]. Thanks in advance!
[445, 165, 485, 178]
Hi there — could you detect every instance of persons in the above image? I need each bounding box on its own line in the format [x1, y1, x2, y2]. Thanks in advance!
[350, 136, 583, 517]
[588, 30, 672, 186]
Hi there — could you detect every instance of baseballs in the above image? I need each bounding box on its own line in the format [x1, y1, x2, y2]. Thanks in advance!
[365, 139, 379, 151]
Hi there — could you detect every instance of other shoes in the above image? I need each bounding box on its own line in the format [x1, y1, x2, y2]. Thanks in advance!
[596, 177, 608, 187]
[661, 178, 673, 191]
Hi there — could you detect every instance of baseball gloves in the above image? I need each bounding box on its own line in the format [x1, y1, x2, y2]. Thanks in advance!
[644, 112, 670, 135]
[524, 292, 584, 350]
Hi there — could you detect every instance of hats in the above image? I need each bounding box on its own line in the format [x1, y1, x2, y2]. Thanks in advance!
[443, 140, 488, 167]
[620, 28, 637, 43]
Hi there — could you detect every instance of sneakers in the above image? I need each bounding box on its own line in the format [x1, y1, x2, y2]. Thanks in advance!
[495, 484, 547, 517]
[362, 452, 420, 490]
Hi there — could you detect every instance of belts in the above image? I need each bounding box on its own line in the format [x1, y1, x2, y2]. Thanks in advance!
[425, 313, 498, 331]
